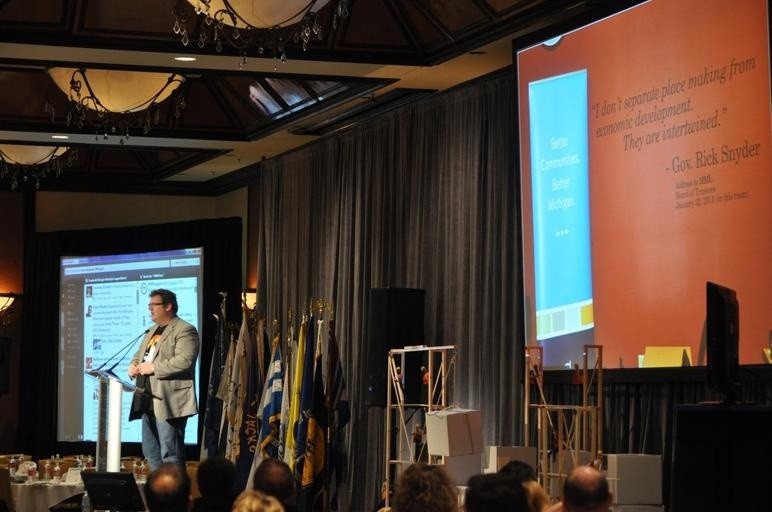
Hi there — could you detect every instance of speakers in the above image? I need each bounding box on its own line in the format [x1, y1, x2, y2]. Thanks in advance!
[367, 286, 427, 406]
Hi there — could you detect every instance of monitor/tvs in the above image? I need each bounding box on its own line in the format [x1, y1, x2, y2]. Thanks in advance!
[705, 281, 741, 403]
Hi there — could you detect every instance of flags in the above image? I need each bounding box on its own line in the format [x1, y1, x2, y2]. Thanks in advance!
[200, 291, 351, 512]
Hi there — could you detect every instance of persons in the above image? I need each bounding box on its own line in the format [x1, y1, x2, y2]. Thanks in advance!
[87, 287, 92, 297]
[139, 456, 299, 512]
[126, 289, 201, 496]
[86, 305, 92, 317]
[87, 358, 92, 368]
[388, 459, 614, 512]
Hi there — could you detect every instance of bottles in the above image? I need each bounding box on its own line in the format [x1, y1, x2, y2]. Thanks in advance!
[10, 453, 147, 486]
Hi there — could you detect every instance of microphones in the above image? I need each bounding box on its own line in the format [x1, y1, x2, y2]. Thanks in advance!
[94, 329, 152, 377]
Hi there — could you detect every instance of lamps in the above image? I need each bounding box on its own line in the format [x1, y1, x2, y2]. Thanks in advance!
[2, 141, 80, 188]
[169, 0, 345, 72]
[45, 64, 188, 144]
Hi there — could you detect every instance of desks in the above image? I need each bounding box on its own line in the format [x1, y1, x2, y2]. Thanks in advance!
[9, 479, 84, 511]
